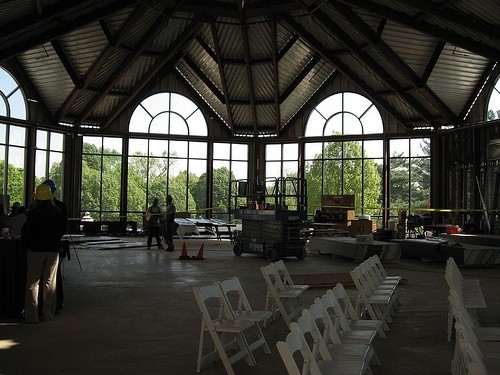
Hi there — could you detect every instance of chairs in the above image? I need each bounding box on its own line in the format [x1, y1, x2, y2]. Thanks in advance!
[191, 254, 402, 375]
[444, 256, 500, 375]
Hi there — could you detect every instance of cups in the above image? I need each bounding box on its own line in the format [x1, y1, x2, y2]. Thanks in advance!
[2, 228, 10, 239]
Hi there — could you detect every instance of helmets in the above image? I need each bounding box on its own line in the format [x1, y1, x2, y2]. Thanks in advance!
[43, 180, 56, 190]
[11, 202, 20, 208]
[165, 195, 173, 200]
[33, 184, 53, 200]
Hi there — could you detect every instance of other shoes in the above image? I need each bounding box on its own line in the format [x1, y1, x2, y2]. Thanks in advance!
[158, 246, 164, 249]
[147, 246, 150, 250]
[166, 247, 174, 252]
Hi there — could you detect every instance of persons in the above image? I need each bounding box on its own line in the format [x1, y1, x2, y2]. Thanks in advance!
[0, 180, 68, 324]
[314, 208, 345, 223]
[145, 195, 176, 253]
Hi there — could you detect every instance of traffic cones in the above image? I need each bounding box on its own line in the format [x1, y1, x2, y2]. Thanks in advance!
[195, 242, 205, 260]
[179, 241, 190, 261]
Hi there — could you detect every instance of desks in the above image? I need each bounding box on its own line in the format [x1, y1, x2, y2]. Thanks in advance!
[173, 217, 236, 242]
[59, 234, 85, 271]
[0, 236, 64, 319]
